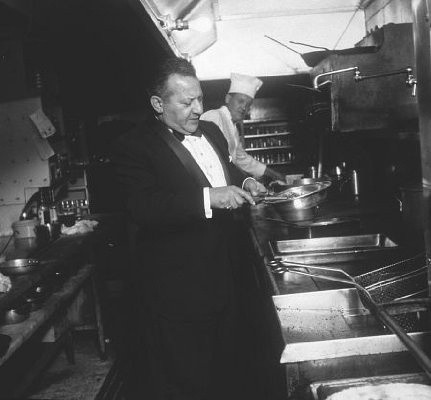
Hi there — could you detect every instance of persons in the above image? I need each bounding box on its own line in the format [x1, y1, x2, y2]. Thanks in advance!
[109, 55, 275, 400]
[199, 72, 287, 183]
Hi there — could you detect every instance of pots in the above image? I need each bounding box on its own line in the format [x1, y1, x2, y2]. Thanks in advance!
[0, 259, 40, 274]
[11, 219, 40, 240]
[251, 181, 332, 222]
[264, 34, 377, 67]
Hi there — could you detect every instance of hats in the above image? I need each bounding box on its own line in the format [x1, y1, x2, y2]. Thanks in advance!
[226, 70, 263, 99]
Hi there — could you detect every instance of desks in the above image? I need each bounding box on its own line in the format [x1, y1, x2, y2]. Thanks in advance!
[1, 235, 106, 400]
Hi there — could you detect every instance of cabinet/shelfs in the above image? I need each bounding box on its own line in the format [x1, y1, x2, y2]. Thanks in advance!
[242, 117, 292, 166]
[41, 76, 88, 201]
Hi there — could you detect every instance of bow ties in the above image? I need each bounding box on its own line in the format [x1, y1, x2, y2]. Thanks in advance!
[172, 128, 203, 141]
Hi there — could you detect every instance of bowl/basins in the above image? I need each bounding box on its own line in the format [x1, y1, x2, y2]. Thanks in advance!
[2, 301, 31, 327]
[25, 283, 50, 313]
